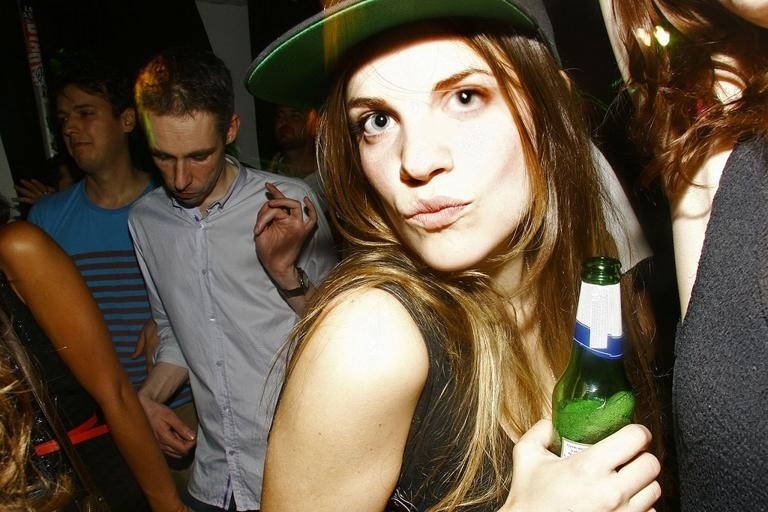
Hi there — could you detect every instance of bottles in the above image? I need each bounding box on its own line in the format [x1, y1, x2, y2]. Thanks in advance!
[242, 0, 567, 114]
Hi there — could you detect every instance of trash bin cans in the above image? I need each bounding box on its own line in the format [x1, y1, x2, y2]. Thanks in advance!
[277, 266, 311, 300]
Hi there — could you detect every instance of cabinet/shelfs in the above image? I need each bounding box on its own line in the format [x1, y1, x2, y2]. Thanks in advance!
[552, 256, 635, 458]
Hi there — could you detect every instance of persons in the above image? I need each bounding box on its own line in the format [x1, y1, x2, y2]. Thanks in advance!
[264, 106, 318, 177]
[595, 0, 768, 511]
[241, 0, 677, 512]
[134, 45, 341, 512]
[9, 142, 80, 206]
[0, 199, 191, 512]
[28, 66, 218, 512]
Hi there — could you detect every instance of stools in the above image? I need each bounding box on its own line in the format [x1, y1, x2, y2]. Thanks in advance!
[28, 412, 110, 456]
